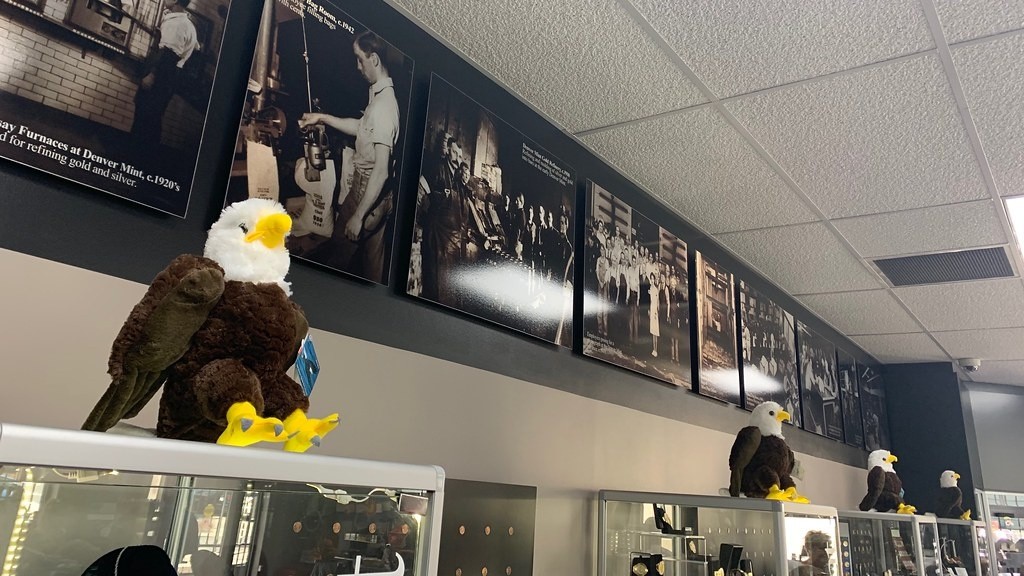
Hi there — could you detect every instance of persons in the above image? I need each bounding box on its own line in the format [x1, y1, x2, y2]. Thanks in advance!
[586, 217, 689, 365]
[125, 0, 202, 163]
[800, 340, 839, 404]
[741, 318, 800, 426]
[298, 29, 400, 269]
[411, 136, 574, 296]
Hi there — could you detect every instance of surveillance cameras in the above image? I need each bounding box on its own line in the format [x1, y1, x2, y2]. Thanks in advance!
[958, 359, 981, 373]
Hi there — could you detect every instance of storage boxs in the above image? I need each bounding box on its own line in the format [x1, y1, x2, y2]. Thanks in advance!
[0, 423, 447, 576]
[595, 489, 998, 576]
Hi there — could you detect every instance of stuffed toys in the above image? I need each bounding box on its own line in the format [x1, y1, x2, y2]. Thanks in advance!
[84, 194, 341, 451]
[934, 469, 973, 520]
[729, 401, 810, 504]
[859, 449, 918, 513]
